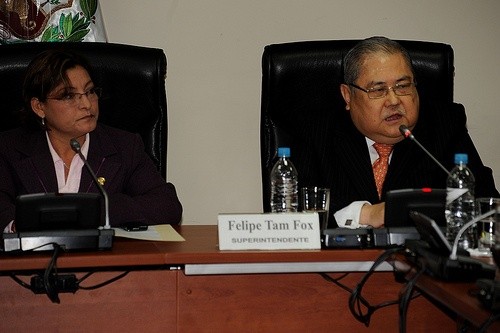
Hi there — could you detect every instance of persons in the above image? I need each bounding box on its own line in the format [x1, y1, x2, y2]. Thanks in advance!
[292, 37, 500, 231]
[0, 49, 183, 234]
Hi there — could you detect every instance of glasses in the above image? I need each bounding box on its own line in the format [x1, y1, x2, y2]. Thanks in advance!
[350, 79, 416, 99]
[46, 87, 101, 104]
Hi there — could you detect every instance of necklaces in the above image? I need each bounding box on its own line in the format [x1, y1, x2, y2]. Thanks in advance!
[63, 163, 69, 170]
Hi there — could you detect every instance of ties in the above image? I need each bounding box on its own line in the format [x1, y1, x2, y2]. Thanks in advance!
[371, 143, 393, 199]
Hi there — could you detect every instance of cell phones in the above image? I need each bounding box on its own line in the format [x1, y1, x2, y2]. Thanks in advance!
[119, 221, 148, 232]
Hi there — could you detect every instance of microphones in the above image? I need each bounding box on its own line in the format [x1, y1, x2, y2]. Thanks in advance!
[383, 124, 474, 227]
[1, 137, 115, 255]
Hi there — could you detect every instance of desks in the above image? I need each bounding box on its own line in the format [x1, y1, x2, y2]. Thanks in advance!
[0, 223, 500, 333]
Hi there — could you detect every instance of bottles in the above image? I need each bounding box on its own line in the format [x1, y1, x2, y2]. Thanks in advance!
[269, 148, 298, 213]
[445, 153, 476, 251]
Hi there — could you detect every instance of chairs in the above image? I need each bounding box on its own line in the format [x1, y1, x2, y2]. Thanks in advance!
[259, 39, 455, 214]
[0, 42, 170, 219]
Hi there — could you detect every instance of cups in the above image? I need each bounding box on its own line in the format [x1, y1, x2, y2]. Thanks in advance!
[301, 188, 330, 243]
[475, 198, 500, 253]
